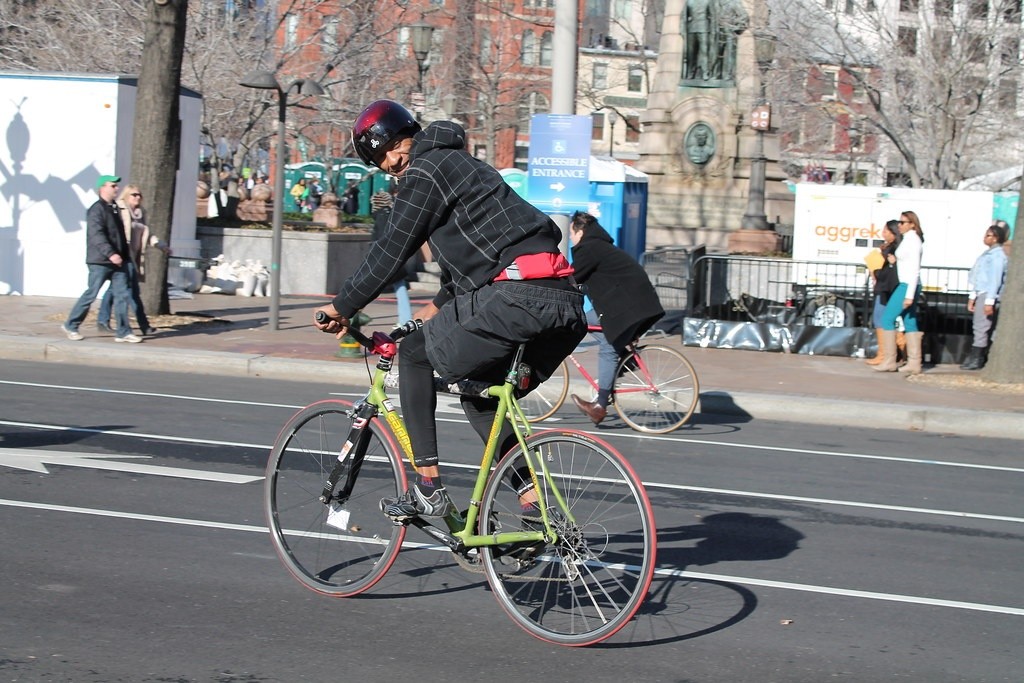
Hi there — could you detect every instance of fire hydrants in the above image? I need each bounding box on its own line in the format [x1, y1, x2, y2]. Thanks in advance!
[330, 311, 371, 358]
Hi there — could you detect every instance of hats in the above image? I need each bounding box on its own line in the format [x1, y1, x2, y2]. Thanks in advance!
[95, 176, 121, 188]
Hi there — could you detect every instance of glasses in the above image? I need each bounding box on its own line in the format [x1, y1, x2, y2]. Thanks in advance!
[103, 184, 117, 189]
[130, 192, 141, 197]
[985, 233, 997, 238]
[898, 220, 914, 225]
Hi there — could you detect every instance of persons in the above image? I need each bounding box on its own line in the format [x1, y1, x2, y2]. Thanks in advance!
[313, 99, 587, 533]
[60, 176, 142, 342]
[961, 225, 1007, 369]
[680, 0, 749, 81]
[866, 213, 924, 372]
[290, 178, 323, 213]
[238, 174, 268, 192]
[370, 185, 423, 329]
[688, 128, 711, 161]
[343, 180, 360, 213]
[96, 184, 173, 334]
[199, 156, 231, 172]
[570, 212, 666, 424]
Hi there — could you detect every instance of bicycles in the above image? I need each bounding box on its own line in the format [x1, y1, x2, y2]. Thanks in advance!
[505, 324, 700, 435]
[264, 310, 657, 647]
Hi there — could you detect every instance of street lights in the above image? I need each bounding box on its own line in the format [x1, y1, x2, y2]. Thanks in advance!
[238, 69, 326, 331]
[406, 14, 435, 122]
[608, 110, 618, 158]
[741, 29, 778, 231]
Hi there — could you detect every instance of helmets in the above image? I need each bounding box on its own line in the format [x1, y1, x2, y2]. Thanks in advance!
[352, 98, 422, 166]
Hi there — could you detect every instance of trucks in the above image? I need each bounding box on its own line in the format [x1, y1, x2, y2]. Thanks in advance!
[789, 182, 1019, 328]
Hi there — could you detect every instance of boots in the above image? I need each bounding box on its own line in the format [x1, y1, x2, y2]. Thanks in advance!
[959, 344, 985, 371]
[864, 329, 888, 365]
[892, 328, 911, 368]
[897, 331, 924, 373]
[871, 328, 899, 373]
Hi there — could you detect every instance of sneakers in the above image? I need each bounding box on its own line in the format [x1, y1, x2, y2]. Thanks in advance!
[378, 483, 450, 517]
[61, 324, 85, 341]
[115, 331, 143, 343]
[502, 506, 562, 556]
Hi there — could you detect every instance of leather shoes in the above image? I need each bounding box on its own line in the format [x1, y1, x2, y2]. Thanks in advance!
[572, 394, 607, 426]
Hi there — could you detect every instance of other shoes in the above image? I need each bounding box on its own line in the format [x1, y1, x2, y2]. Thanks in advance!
[143, 326, 156, 336]
[97, 322, 116, 333]
